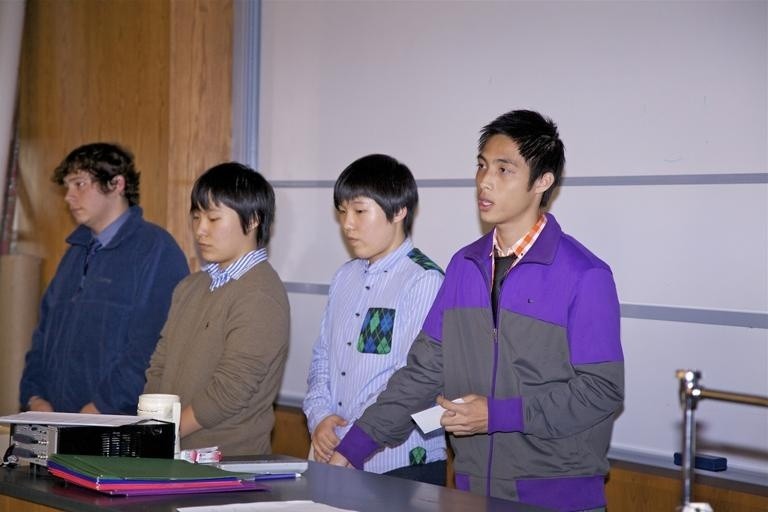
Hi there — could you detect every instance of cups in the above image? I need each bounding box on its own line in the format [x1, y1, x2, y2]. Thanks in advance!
[137, 393, 182, 459]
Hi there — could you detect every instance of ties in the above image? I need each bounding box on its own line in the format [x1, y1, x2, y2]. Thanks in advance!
[490, 255, 517, 329]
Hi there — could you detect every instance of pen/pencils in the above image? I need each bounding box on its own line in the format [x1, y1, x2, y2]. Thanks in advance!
[252, 472, 301, 481]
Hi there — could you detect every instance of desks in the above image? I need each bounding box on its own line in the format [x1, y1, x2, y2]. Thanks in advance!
[0, 453, 557, 512]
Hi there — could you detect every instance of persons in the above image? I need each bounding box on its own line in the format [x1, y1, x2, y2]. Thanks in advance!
[18, 143, 188, 416]
[139, 163, 292, 457]
[326, 110, 624, 512]
[303, 155, 448, 486]
